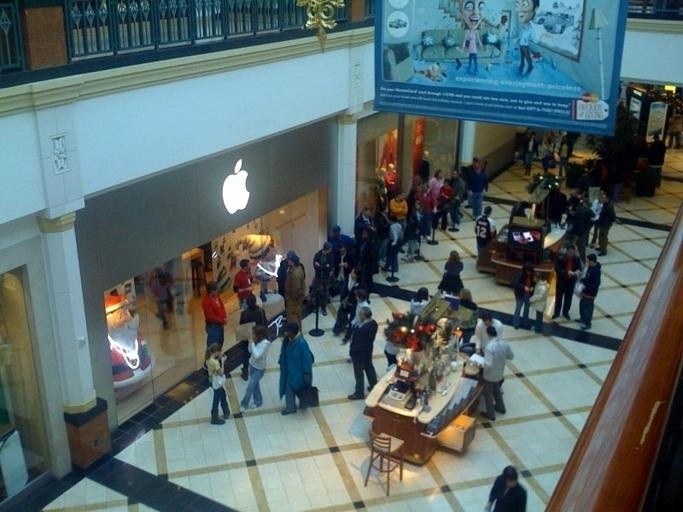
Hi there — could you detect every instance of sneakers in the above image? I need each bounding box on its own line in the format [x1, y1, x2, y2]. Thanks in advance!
[240, 404, 259, 412]
[321, 309, 327, 316]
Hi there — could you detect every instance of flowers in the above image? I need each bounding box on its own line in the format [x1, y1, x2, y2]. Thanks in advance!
[384, 311, 437, 352]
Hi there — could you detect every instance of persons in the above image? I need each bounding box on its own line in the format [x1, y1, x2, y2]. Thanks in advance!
[203, 341, 230, 425]
[346, 305, 378, 400]
[202, 280, 226, 372]
[231, 259, 253, 311]
[148, 266, 170, 329]
[238, 295, 266, 380]
[276, 84, 683, 369]
[277, 323, 315, 415]
[474, 325, 516, 421]
[485, 464, 527, 512]
[237, 324, 271, 411]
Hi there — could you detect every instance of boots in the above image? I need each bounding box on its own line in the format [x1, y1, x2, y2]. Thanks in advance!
[222, 403, 230, 418]
[241, 368, 248, 380]
[211, 410, 225, 424]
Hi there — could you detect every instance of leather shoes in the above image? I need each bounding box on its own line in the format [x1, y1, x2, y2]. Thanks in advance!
[482, 405, 506, 420]
[590, 244, 607, 256]
[348, 394, 364, 399]
[282, 409, 296, 415]
[552, 314, 591, 329]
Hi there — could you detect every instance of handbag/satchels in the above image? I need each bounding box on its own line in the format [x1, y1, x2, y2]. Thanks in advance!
[299, 387, 317, 408]
[236, 322, 255, 341]
[212, 374, 226, 389]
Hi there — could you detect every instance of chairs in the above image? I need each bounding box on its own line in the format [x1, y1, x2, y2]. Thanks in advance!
[363, 427, 405, 497]
